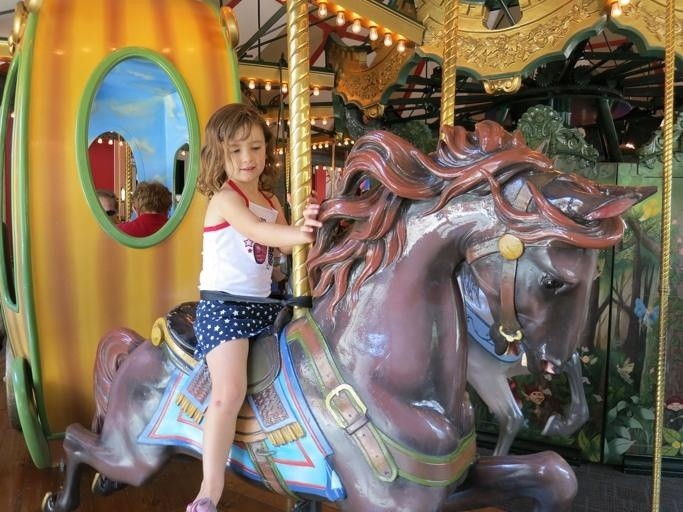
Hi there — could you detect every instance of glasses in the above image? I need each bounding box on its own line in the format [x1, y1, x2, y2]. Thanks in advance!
[106, 211, 117, 216]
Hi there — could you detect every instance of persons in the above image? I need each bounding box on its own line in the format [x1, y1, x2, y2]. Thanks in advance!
[182, 102, 324, 512]
[116, 179, 173, 238]
[95, 190, 119, 222]
